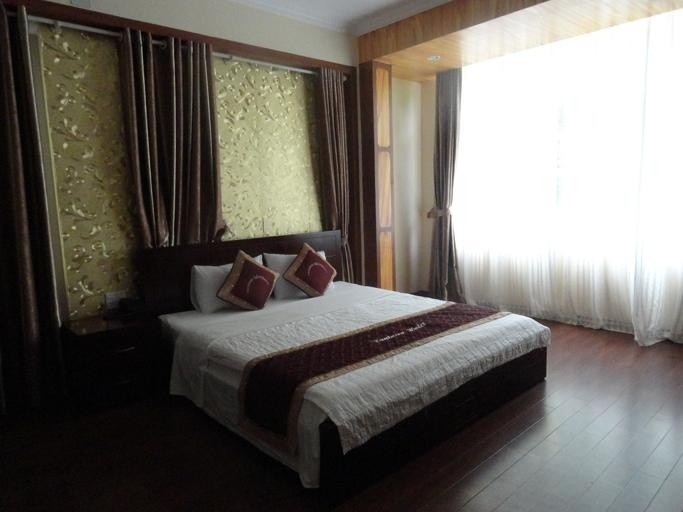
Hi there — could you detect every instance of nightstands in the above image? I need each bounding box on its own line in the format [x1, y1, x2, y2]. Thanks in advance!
[62, 314, 149, 413]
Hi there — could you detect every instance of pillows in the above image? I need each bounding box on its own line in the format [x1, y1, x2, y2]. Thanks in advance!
[263, 251, 335, 298]
[215, 250, 285, 312]
[283, 242, 338, 298]
[191, 254, 264, 313]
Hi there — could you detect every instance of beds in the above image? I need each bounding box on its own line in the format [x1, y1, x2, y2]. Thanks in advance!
[136, 229, 551, 512]
